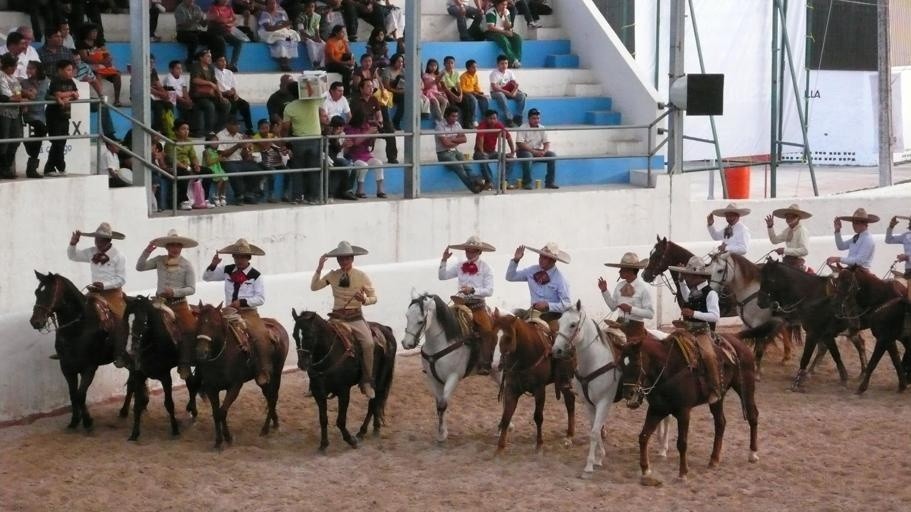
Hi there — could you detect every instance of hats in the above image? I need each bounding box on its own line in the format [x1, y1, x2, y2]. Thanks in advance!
[710, 203, 751, 217]
[148, 230, 200, 249]
[772, 203, 811, 221]
[524, 243, 570, 263]
[834, 208, 878, 223]
[218, 239, 266, 256]
[448, 237, 496, 254]
[194, 48, 210, 55]
[77, 223, 127, 241]
[603, 252, 649, 269]
[896, 214, 909, 221]
[324, 241, 368, 258]
[667, 257, 713, 276]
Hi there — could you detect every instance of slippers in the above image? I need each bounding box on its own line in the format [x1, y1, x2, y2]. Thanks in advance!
[378, 192, 387, 199]
[354, 192, 366, 197]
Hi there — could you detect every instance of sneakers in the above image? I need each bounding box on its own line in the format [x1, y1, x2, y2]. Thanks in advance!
[235, 196, 244, 206]
[178, 200, 191, 210]
[500, 182, 514, 189]
[245, 197, 258, 204]
[203, 200, 215, 209]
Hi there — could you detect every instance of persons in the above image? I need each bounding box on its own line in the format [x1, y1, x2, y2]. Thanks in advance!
[204, 136, 229, 207]
[49, 222, 130, 368]
[505, 241, 572, 391]
[353, 53, 388, 102]
[667, 255, 722, 404]
[826, 208, 880, 272]
[325, 24, 355, 96]
[129, 54, 174, 131]
[382, 53, 405, 131]
[0, 31, 28, 79]
[257, 0, 301, 71]
[515, 108, 559, 190]
[472, 109, 515, 191]
[175, 0, 213, 72]
[44, 61, 80, 177]
[136, 229, 196, 379]
[296, 1, 326, 69]
[149, 0, 161, 43]
[20, 60, 48, 179]
[70, 44, 121, 142]
[202, 238, 271, 385]
[70, 0, 105, 42]
[433, 106, 484, 193]
[151, 129, 167, 211]
[706, 202, 751, 257]
[58, 21, 76, 48]
[78, 25, 121, 106]
[597, 252, 654, 329]
[419, 59, 447, 123]
[208, 0, 242, 72]
[266, 74, 293, 128]
[764, 203, 813, 270]
[440, 56, 462, 123]
[119, 129, 132, 168]
[460, 59, 488, 127]
[397, 27, 405, 58]
[516, 0, 543, 28]
[482, 0, 522, 67]
[351, 79, 399, 163]
[217, 118, 262, 202]
[254, 119, 293, 200]
[164, 123, 215, 210]
[365, 28, 390, 64]
[214, 55, 253, 130]
[36, 29, 74, 63]
[189, 49, 231, 134]
[489, 54, 526, 127]
[330, 0, 403, 43]
[304, 241, 378, 399]
[283, 81, 327, 202]
[102, 138, 128, 188]
[163, 59, 193, 113]
[0, 51, 22, 179]
[445, 0, 484, 41]
[320, 81, 352, 126]
[422, 235, 496, 375]
[885, 214, 911, 281]
[318, 108, 388, 201]
[15, 25, 41, 63]
[483, 0, 517, 27]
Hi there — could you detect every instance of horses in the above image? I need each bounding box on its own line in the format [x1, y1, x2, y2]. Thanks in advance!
[121, 293, 204, 447]
[638, 235, 760, 347]
[402, 288, 518, 444]
[603, 328, 762, 484]
[707, 252, 806, 370]
[823, 261, 909, 395]
[194, 302, 289, 454]
[292, 309, 397, 451]
[755, 258, 866, 388]
[491, 307, 603, 462]
[552, 297, 671, 478]
[29, 269, 136, 435]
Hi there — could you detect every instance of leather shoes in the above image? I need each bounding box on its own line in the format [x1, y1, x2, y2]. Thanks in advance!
[479, 365, 492, 377]
[708, 390, 723, 404]
[545, 182, 559, 188]
[363, 382, 376, 399]
[522, 184, 533, 190]
[113, 354, 130, 367]
[178, 364, 192, 380]
[259, 372, 270, 385]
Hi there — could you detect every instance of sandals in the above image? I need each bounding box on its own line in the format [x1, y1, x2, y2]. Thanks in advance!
[212, 195, 220, 206]
[221, 195, 227, 207]
